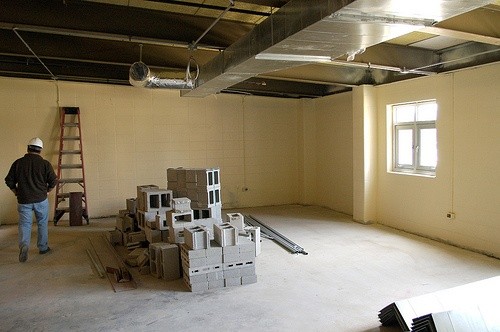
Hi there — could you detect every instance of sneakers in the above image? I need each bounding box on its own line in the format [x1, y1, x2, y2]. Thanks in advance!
[19, 245, 28, 263]
[39, 247, 50, 254]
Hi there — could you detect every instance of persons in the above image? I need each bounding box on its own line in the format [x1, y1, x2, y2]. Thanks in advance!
[5, 138, 58, 263]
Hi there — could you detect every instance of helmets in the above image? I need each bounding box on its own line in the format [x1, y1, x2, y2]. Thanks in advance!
[28, 137, 43, 149]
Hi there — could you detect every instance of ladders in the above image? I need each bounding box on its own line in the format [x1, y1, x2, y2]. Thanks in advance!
[53, 106, 90, 226]
